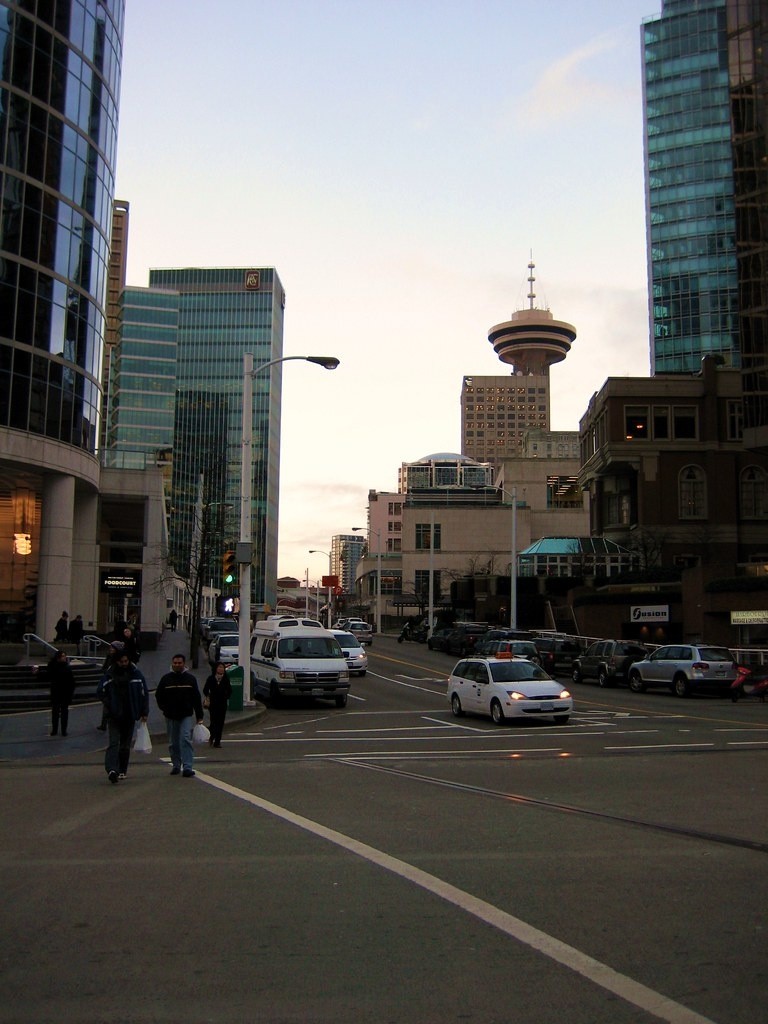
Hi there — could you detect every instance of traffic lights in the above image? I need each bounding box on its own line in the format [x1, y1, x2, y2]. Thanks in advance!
[224, 552, 236, 583]
[215, 595, 235, 613]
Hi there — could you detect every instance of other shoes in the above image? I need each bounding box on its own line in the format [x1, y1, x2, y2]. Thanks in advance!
[213, 742, 222, 748]
[96, 725, 107, 731]
[62, 732, 67, 737]
[50, 730, 57, 736]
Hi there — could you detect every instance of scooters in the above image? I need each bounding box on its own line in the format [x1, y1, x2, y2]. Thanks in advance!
[730, 663, 768, 705]
[396, 621, 430, 645]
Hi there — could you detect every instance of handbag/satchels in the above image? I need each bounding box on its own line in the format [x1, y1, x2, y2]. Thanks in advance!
[133, 720, 153, 755]
[202, 696, 210, 709]
[191, 723, 211, 747]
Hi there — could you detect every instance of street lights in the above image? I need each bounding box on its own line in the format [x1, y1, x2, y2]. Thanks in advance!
[239, 351, 340, 706]
[309, 550, 333, 628]
[469, 483, 516, 629]
[302, 579, 319, 620]
[352, 527, 382, 635]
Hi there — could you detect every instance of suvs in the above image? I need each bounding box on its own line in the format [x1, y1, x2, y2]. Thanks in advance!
[628, 641, 739, 698]
[429, 621, 579, 676]
[571, 639, 650, 687]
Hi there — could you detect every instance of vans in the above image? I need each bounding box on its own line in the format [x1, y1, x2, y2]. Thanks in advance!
[250, 619, 352, 711]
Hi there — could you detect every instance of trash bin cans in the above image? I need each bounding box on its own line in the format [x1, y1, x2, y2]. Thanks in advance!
[225, 664, 244, 711]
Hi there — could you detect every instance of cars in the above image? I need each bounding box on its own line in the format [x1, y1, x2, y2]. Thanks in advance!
[324, 616, 372, 677]
[198, 615, 240, 666]
[447, 656, 573, 727]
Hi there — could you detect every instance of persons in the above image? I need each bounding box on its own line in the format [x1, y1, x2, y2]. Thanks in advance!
[169, 609, 177, 632]
[155, 654, 204, 777]
[407, 615, 415, 636]
[46, 649, 75, 737]
[97, 641, 125, 731]
[638, 623, 664, 646]
[55, 611, 83, 656]
[203, 661, 232, 748]
[114, 614, 141, 665]
[97, 650, 150, 784]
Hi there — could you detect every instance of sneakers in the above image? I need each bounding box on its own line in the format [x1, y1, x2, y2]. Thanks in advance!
[108, 770, 118, 784]
[170, 768, 181, 775]
[182, 770, 195, 778]
[118, 768, 127, 779]
